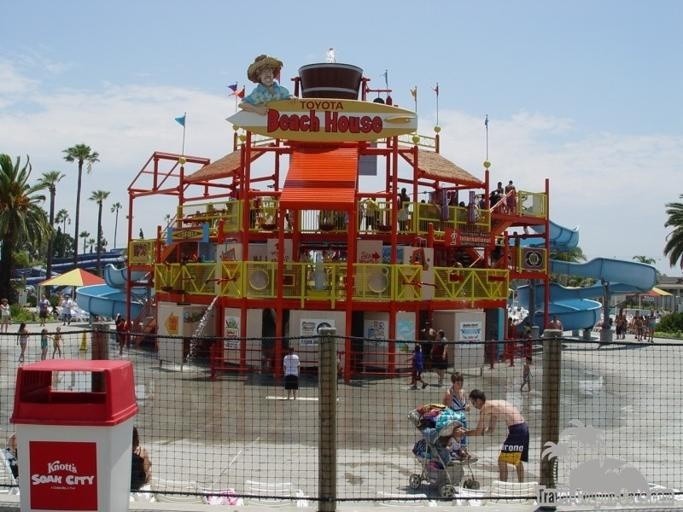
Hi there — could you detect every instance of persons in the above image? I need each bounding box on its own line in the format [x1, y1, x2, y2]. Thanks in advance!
[6, 433, 19, 479]
[130, 426, 156, 502]
[61, 294, 73, 326]
[442, 372, 530, 482]
[37, 294, 51, 326]
[506, 316, 531, 353]
[520, 356, 533, 392]
[615, 309, 662, 343]
[41, 326, 64, 361]
[0, 298, 10, 333]
[397, 187, 410, 232]
[283, 347, 301, 400]
[550, 315, 563, 336]
[406, 320, 448, 390]
[361, 195, 379, 234]
[115, 313, 142, 355]
[451, 260, 466, 297]
[16, 322, 29, 363]
[476, 180, 516, 215]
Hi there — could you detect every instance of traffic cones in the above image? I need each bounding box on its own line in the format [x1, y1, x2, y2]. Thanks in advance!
[78, 329, 88, 350]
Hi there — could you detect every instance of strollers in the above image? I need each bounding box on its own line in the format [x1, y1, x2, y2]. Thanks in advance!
[406, 402, 481, 499]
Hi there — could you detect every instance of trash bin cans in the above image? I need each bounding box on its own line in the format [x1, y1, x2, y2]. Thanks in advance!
[9, 359, 140, 512]
